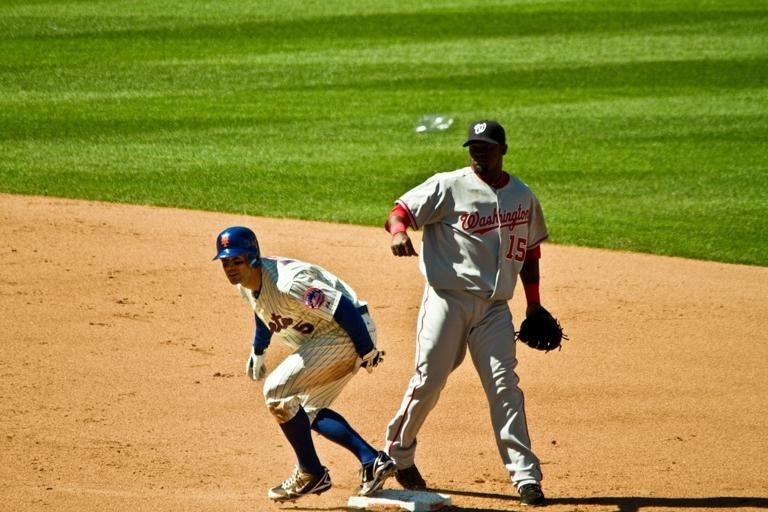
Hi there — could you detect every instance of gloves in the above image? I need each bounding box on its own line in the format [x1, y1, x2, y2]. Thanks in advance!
[352, 348, 384, 374]
[246, 345, 266, 381]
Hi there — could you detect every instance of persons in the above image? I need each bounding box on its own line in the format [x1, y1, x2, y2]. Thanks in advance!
[213, 226, 396, 503]
[382, 119, 562, 504]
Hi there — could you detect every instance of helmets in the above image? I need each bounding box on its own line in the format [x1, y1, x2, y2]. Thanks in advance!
[212, 227, 262, 268]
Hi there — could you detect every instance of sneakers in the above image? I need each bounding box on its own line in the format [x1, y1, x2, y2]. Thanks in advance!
[358, 451, 397, 496]
[268, 466, 331, 501]
[395, 465, 426, 490]
[519, 483, 544, 504]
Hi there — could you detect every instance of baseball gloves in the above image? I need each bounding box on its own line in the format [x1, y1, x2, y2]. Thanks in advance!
[520, 306, 562, 350]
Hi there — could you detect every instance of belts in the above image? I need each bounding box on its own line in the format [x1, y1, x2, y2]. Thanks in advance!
[357, 305, 368, 313]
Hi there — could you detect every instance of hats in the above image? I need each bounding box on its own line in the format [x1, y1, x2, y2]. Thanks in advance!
[463, 122, 505, 148]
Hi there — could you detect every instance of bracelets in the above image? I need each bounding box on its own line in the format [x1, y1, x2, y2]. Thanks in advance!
[524, 282, 542, 304]
[390, 221, 407, 235]
